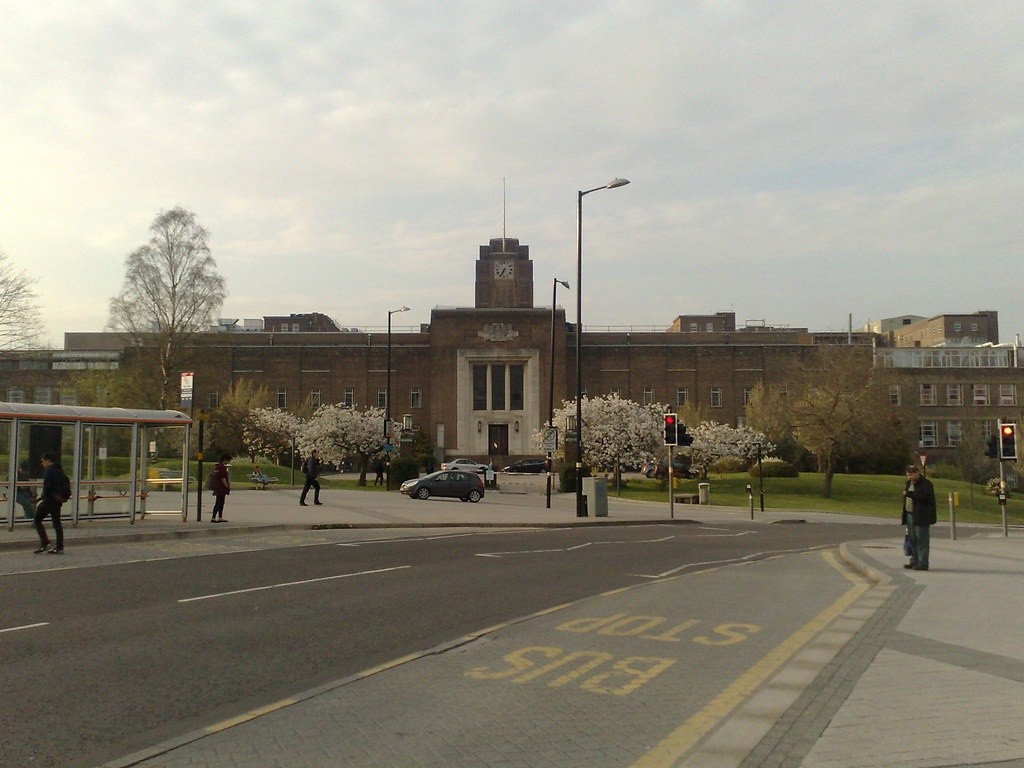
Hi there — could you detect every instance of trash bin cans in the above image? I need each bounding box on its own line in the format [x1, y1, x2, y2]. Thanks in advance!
[483, 466, 497, 489]
[582, 477, 608, 517]
[698, 483, 711, 501]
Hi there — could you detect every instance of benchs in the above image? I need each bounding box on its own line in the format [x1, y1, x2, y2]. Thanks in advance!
[672, 494, 699, 505]
[0, 493, 149, 509]
[157, 469, 196, 492]
[247, 473, 279, 491]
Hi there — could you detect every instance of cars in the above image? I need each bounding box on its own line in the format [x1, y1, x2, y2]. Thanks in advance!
[440, 458, 495, 474]
[501, 458, 548, 475]
[399, 469, 485, 503]
[641, 456, 705, 480]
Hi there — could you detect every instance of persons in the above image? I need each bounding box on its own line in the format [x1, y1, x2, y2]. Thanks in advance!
[33, 452, 67, 554]
[5, 460, 38, 519]
[374, 461, 385, 486]
[901, 464, 937, 571]
[300, 449, 322, 506]
[425, 461, 434, 475]
[211, 454, 234, 523]
[252, 466, 268, 485]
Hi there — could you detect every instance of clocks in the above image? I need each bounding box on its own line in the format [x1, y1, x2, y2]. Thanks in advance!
[493, 259, 514, 280]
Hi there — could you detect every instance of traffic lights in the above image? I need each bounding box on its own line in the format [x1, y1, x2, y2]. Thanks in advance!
[1000, 424, 1018, 459]
[677, 423, 694, 446]
[663, 413, 678, 446]
[984, 435, 998, 458]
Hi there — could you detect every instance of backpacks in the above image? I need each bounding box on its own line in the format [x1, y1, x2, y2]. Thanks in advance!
[301, 461, 309, 474]
[51, 471, 71, 504]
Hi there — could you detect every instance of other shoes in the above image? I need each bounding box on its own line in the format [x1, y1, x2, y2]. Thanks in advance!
[34, 543, 53, 554]
[218, 519, 228, 522]
[48, 547, 65, 554]
[300, 503, 308, 506]
[904, 562, 929, 570]
[313, 502, 322, 505]
[211, 519, 219, 523]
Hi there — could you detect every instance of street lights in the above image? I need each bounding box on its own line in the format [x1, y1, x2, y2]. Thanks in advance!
[575, 176, 632, 518]
[545, 277, 572, 508]
[386, 306, 411, 492]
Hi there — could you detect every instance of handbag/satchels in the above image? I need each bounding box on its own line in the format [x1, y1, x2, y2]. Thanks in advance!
[903, 533, 913, 556]
[205, 474, 216, 491]
